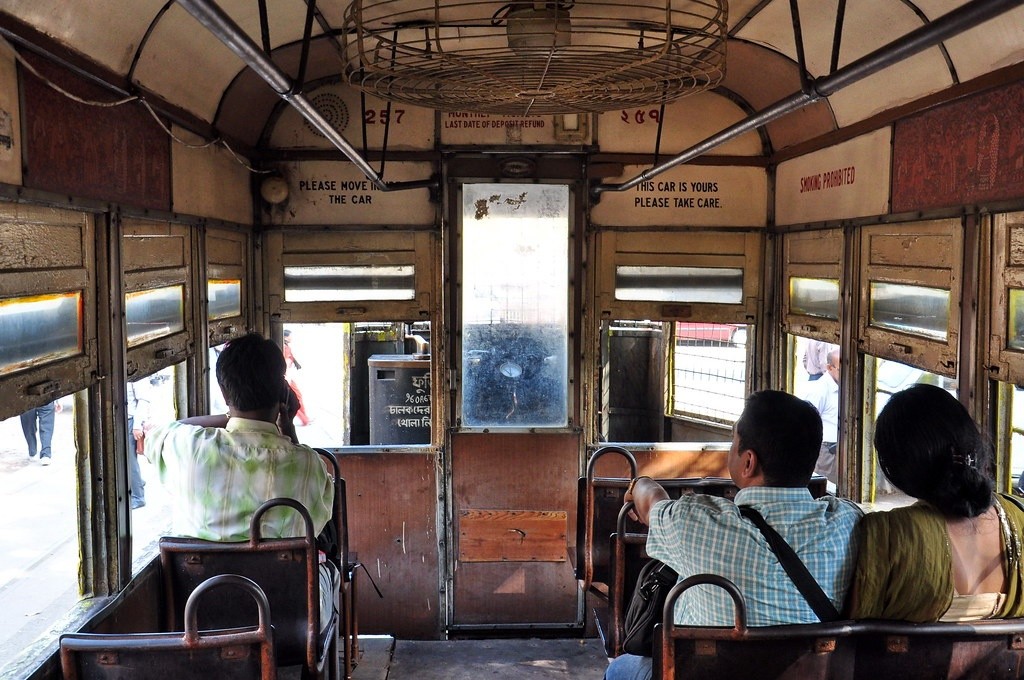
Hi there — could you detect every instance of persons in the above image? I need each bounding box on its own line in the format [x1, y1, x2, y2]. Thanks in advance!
[20, 398, 63, 466]
[848, 384, 1024, 680]
[127, 378, 151, 510]
[284, 329, 316, 427]
[604, 389, 864, 680]
[144, 333, 334, 634]
[803, 339, 840, 487]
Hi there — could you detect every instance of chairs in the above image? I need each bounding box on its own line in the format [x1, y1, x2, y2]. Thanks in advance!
[58, 447, 361, 679]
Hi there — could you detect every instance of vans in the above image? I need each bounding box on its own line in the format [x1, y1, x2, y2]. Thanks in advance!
[676, 321, 747, 348]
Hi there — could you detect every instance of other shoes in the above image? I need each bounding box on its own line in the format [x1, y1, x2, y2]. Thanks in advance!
[29, 453, 37, 460]
[40, 457, 50, 466]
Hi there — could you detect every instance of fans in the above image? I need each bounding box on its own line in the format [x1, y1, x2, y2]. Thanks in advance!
[341, 0, 729, 119]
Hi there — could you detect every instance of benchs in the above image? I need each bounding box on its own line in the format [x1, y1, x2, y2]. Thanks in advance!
[565, 447, 1024, 680]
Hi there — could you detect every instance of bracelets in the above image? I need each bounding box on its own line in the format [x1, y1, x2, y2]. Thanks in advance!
[629, 476, 652, 495]
[225, 410, 230, 418]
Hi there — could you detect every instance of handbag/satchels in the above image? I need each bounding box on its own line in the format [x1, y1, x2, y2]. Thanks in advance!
[137, 421, 150, 454]
[622, 559, 680, 657]
